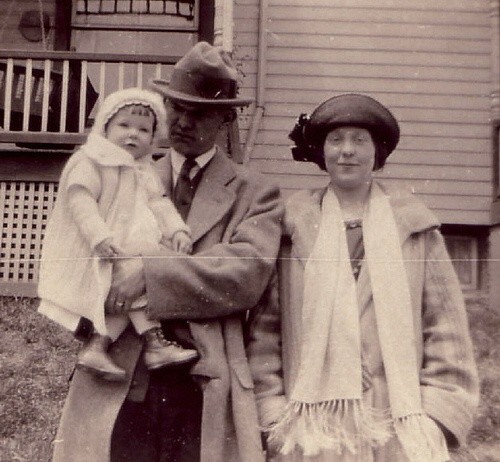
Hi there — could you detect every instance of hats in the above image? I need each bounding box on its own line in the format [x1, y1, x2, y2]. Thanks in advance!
[94, 87, 166, 138]
[288, 93, 400, 162]
[151, 41, 253, 105]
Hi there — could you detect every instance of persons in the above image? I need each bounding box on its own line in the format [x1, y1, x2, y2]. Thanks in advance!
[52, 41, 286, 462]
[244, 94, 480, 462]
[37, 87, 199, 380]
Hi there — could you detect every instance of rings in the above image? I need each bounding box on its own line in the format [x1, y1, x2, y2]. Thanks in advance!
[115, 301, 125, 308]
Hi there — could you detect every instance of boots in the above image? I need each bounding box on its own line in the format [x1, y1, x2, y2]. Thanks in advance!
[75, 333, 126, 381]
[142, 326, 197, 369]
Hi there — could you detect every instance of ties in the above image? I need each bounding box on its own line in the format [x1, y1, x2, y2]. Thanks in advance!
[174, 158, 197, 207]
